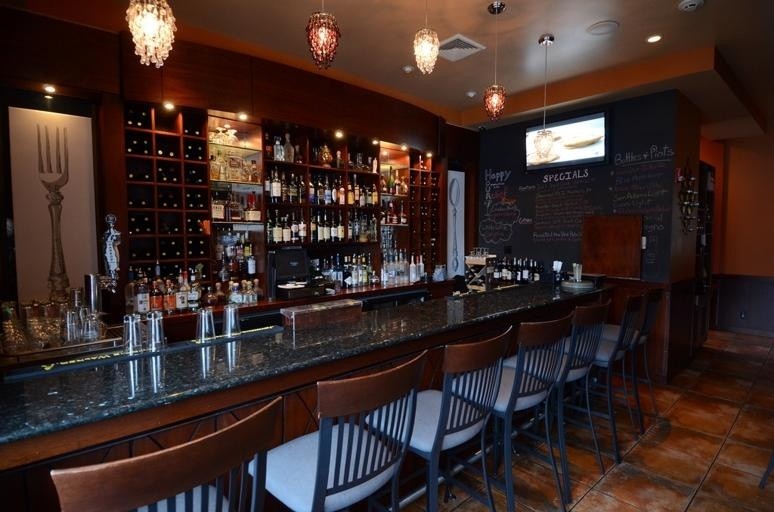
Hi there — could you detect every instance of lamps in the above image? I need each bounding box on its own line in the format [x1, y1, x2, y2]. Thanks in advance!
[119, 0, 181, 72]
[527, 33, 557, 166]
[305, 1, 341, 72]
[406, 0, 443, 78]
[480, 3, 508, 124]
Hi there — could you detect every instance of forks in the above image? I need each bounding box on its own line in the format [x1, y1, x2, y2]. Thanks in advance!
[34, 123, 70, 308]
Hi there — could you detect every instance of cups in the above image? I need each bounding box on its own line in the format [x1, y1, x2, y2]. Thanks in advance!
[198, 348, 216, 381]
[149, 356, 168, 393]
[122, 313, 142, 358]
[145, 309, 162, 358]
[125, 360, 144, 400]
[223, 338, 238, 374]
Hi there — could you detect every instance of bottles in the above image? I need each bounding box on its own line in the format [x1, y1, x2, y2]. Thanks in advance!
[65, 133, 590, 342]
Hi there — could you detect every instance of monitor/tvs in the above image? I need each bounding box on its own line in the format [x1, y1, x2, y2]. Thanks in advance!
[519, 107, 610, 174]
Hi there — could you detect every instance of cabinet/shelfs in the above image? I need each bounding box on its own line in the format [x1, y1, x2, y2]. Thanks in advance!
[115, 98, 212, 292]
[301, 127, 350, 248]
[198, 105, 274, 305]
[258, 110, 309, 249]
[460, 252, 494, 294]
[375, 137, 411, 268]
[342, 130, 380, 243]
[402, 142, 447, 275]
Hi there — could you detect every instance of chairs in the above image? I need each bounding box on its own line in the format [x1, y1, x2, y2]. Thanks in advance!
[583, 282, 666, 442]
[46, 395, 288, 511]
[446, 300, 575, 511]
[555, 285, 658, 471]
[501, 290, 616, 504]
[361, 322, 514, 511]
[242, 347, 432, 511]
